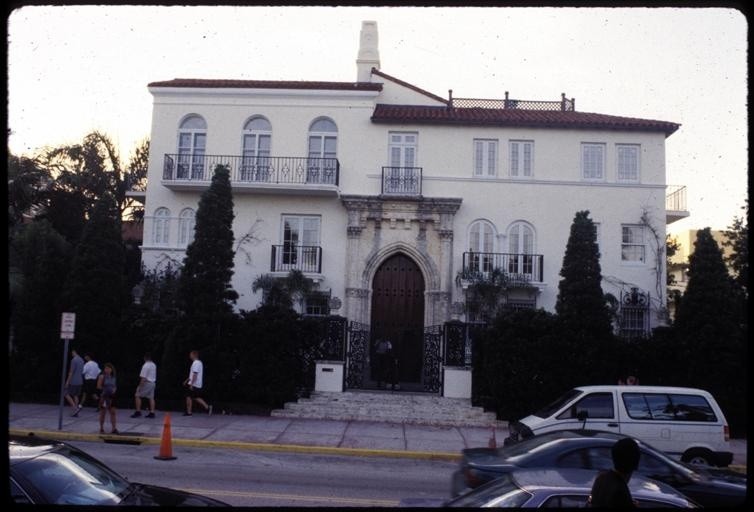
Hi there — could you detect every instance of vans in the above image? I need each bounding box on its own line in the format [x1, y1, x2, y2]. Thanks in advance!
[504, 384, 736, 467]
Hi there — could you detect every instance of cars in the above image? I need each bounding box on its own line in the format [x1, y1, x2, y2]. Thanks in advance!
[450, 428, 747, 511]
[399, 467, 710, 512]
[8, 434, 234, 505]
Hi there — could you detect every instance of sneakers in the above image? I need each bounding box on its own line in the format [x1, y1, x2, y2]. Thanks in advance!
[95, 405, 100, 413]
[112, 428, 120, 435]
[70, 404, 82, 417]
[182, 412, 193, 417]
[145, 412, 156, 418]
[130, 410, 142, 418]
[206, 405, 213, 417]
[100, 428, 104, 433]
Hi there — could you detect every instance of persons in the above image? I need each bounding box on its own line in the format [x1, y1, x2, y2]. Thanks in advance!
[95, 363, 120, 434]
[130, 351, 157, 420]
[374, 333, 392, 387]
[182, 349, 214, 416]
[590, 438, 642, 509]
[617, 376, 640, 385]
[78, 353, 101, 412]
[61, 346, 85, 417]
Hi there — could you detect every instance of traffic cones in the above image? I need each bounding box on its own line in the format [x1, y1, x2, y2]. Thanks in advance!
[153, 411, 178, 460]
[486, 425, 498, 448]
